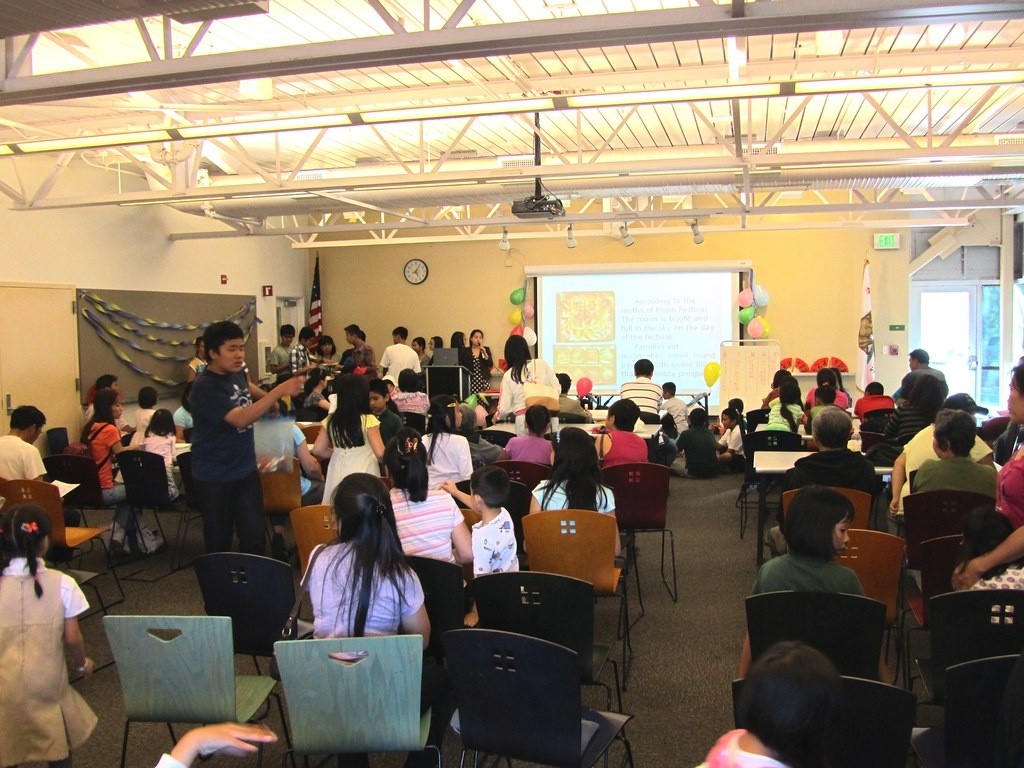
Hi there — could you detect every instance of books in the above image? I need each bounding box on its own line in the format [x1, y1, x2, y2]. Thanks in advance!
[50, 478, 80, 497]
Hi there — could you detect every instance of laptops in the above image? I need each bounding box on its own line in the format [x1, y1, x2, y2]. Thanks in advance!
[433, 348, 458, 366]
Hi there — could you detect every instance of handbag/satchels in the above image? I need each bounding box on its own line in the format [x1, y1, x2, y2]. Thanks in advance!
[523, 359, 560, 417]
[269, 543, 329, 681]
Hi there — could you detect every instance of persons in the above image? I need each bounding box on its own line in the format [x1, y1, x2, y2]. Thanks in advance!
[153, 722, 277, 768]
[736, 392, 998, 686]
[955, 355, 1024, 623]
[180, 318, 325, 614]
[0, 506, 101, 768]
[619, 360, 747, 479]
[302, 373, 648, 768]
[694, 641, 847, 768]
[272, 323, 592, 452]
[759, 348, 948, 453]
[1, 403, 81, 528]
[81, 374, 196, 553]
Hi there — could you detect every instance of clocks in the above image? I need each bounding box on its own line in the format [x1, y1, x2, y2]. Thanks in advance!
[404, 259, 428, 284]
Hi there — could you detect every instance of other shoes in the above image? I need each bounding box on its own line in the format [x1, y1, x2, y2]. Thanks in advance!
[109, 522, 130, 555]
[272, 530, 289, 565]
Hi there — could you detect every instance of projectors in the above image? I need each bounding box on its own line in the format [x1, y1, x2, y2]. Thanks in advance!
[511, 199, 565, 219]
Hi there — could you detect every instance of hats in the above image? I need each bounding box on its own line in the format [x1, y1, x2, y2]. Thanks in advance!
[944, 394, 989, 417]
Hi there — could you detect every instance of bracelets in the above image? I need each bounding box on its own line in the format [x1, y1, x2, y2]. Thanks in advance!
[76, 657, 88, 673]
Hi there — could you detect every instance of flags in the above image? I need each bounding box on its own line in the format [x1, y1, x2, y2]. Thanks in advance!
[309, 256, 322, 355]
[855, 260, 875, 394]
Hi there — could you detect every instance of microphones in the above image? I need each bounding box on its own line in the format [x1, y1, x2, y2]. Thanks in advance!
[478, 342, 481, 347]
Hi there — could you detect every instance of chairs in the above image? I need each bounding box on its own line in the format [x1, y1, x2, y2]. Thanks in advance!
[732, 406, 1024, 768]
[0, 412, 681, 768]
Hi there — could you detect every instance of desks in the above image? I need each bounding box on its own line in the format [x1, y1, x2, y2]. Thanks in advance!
[585, 386, 711, 416]
[478, 390, 587, 411]
[588, 409, 669, 424]
[484, 422, 661, 453]
[752, 450, 901, 570]
[752, 420, 868, 448]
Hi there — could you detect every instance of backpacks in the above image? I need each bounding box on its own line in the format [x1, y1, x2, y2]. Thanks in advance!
[63, 423, 112, 490]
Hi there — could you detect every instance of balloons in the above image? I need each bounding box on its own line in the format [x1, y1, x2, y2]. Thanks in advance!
[509, 288, 536, 347]
[738, 284, 771, 338]
[704, 361, 722, 388]
[463, 394, 488, 427]
[576, 377, 593, 398]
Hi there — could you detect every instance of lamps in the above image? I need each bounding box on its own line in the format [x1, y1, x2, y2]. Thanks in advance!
[620, 221, 634, 245]
[690, 219, 704, 244]
[566, 223, 578, 248]
[499, 225, 511, 250]
